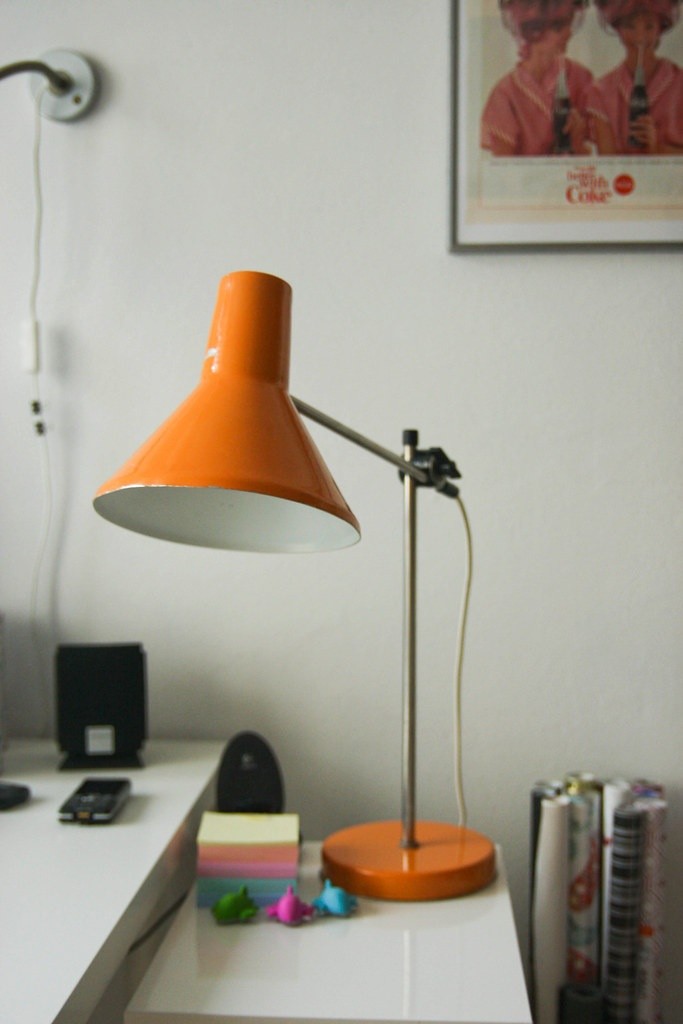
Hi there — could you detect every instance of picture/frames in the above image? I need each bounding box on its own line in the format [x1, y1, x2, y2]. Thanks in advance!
[448, 0, 683, 254]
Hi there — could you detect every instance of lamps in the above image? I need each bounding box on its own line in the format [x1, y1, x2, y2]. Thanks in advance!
[93, 271, 498, 901]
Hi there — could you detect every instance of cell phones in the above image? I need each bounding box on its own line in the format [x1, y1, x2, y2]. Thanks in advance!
[58, 777, 131, 824]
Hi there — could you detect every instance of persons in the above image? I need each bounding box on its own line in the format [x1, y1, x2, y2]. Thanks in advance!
[480, 0, 683, 156]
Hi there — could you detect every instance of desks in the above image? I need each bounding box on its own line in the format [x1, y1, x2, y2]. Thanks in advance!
[122, 839, 534, 1024]
[1, 740, 226, 1022]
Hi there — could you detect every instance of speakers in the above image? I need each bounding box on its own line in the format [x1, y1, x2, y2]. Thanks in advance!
[52, 642, 145, 768]
[216, 732, 286, 814]
